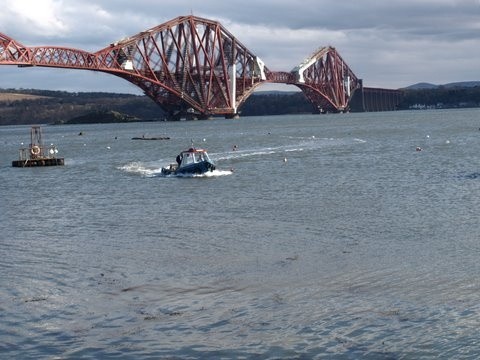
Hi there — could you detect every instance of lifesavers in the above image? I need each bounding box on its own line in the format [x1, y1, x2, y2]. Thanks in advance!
[31, 145, 41, 155]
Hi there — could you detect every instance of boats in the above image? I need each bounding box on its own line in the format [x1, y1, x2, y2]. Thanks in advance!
[161, 139, 215, 176]
[11, 126, 65, 167]
